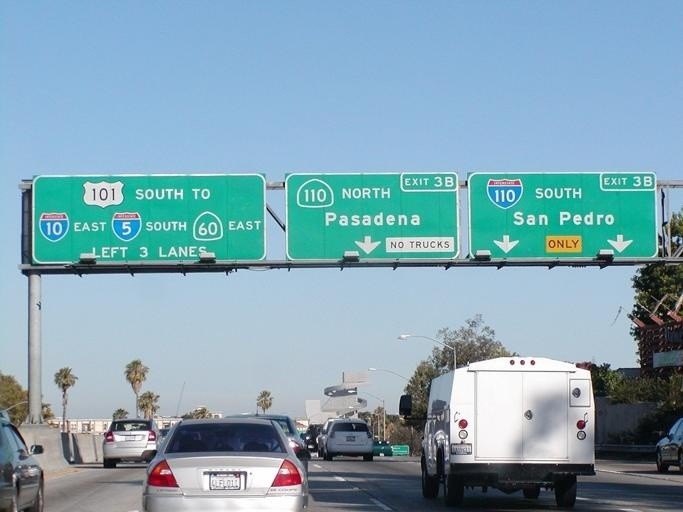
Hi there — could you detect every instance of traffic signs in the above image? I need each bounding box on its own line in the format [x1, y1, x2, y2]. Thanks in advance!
[466, 171, 660, 262]
[31, 172, 268, 265]
[284, 171, 461, 260]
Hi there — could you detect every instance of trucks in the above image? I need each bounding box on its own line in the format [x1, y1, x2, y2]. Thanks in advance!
[400, 357, 596, 512]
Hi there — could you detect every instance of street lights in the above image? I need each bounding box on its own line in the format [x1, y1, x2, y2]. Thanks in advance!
[396, 334, 456, 371]
[347, 389, 386, 442]
[368, 366, 415, 457]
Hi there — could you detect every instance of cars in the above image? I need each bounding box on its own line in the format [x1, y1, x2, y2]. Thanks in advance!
[317, 418, 375, 463]
[141, 416, 313, 512]
[102, 418, 163, 468]
[653, 417, 683, 475]
[300, 423, 324, 454]
[0, 409, 46, 512]
[225, 414, 310, 478]
